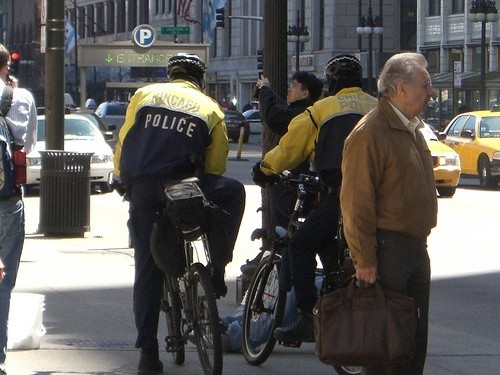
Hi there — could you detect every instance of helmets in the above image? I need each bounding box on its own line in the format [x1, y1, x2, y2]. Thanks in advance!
[324, 52, 362, 87]
[167, 53, 206, 88]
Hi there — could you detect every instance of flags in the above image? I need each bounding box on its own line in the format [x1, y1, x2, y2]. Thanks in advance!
[64, 17, 81, 54]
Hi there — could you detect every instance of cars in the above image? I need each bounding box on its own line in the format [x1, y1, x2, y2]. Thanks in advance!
[243, 110, 262, 134]
[96, 99, 131, 134]
[19, 115, 113, 197]
[71, 110, 117, 153]
[224, 110, 250, 144]
[441, 108, 500, 189]
[419, 121, 462, 199]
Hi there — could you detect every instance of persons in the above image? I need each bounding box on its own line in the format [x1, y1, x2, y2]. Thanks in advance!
[210, 276, 323, 354]
[113, 52, 246, 375]
[255, 71, 323, 257]
[0, 44, 37, 375]
[251, 54, 378, 342]
[340, 52, 439, 375]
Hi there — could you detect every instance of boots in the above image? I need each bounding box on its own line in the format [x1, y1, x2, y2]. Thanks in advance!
[273, 309, 316, 343]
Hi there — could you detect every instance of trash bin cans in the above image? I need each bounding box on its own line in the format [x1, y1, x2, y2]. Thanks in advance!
[38, 150, 94, 237]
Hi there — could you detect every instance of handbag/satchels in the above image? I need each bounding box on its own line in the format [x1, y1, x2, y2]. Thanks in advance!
[312, 273, 424, 368]
[150, 181, 232, 279]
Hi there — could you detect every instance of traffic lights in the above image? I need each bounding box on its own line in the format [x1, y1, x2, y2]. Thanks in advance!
[257, 49, 263, 70]
[11, 52, 20, 74]
[215, 7, 224, 29]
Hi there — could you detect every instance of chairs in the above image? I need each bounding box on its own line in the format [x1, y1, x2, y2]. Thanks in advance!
[481, 123, 489, 133]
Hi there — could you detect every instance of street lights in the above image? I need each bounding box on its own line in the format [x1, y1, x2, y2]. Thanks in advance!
[355, 15, 384, 93]
[287, 25, 310, 71]
[467, 0, 499, 110]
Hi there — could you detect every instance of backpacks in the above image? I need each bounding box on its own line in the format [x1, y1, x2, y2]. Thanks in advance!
[0, 86, 14, 201]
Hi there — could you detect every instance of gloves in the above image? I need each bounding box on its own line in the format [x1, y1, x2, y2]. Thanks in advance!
[250, 160, 280, 187]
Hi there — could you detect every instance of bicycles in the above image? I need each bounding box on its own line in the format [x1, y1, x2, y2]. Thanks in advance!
[158, 165, 228, 375]
[239, 169, 366, 375]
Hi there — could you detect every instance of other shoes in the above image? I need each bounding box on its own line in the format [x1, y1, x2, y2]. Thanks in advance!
[137, 344, 163, 375]
[0, 364, 7, 375]
[205, 256, 229, 297]
